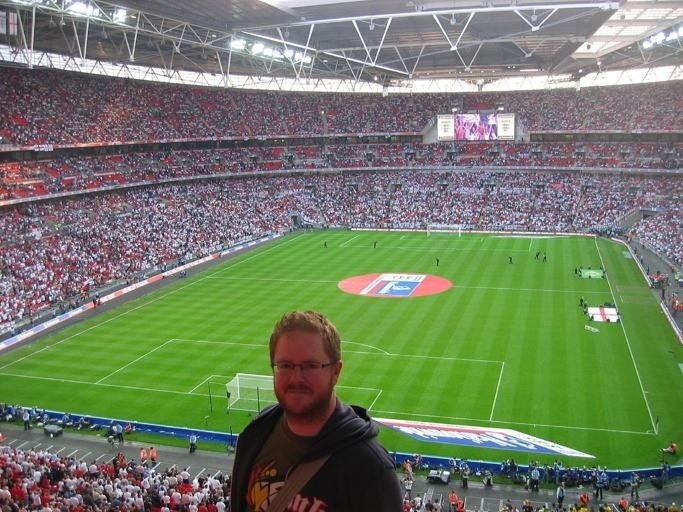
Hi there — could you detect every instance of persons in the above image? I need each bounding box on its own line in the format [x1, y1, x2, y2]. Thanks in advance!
[228, 310, 405, 512]
[445, 91, 502, 112]
[499, 80, 683, 324]
[1, 401, 230, 512]
[455, 114, 498, 140]
[442, 141, 512, 231]
[402, 442, 683, 512]
[1, 69, 448, 338]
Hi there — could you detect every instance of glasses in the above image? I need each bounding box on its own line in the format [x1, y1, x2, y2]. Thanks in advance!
[271, 359, 335, 372]
